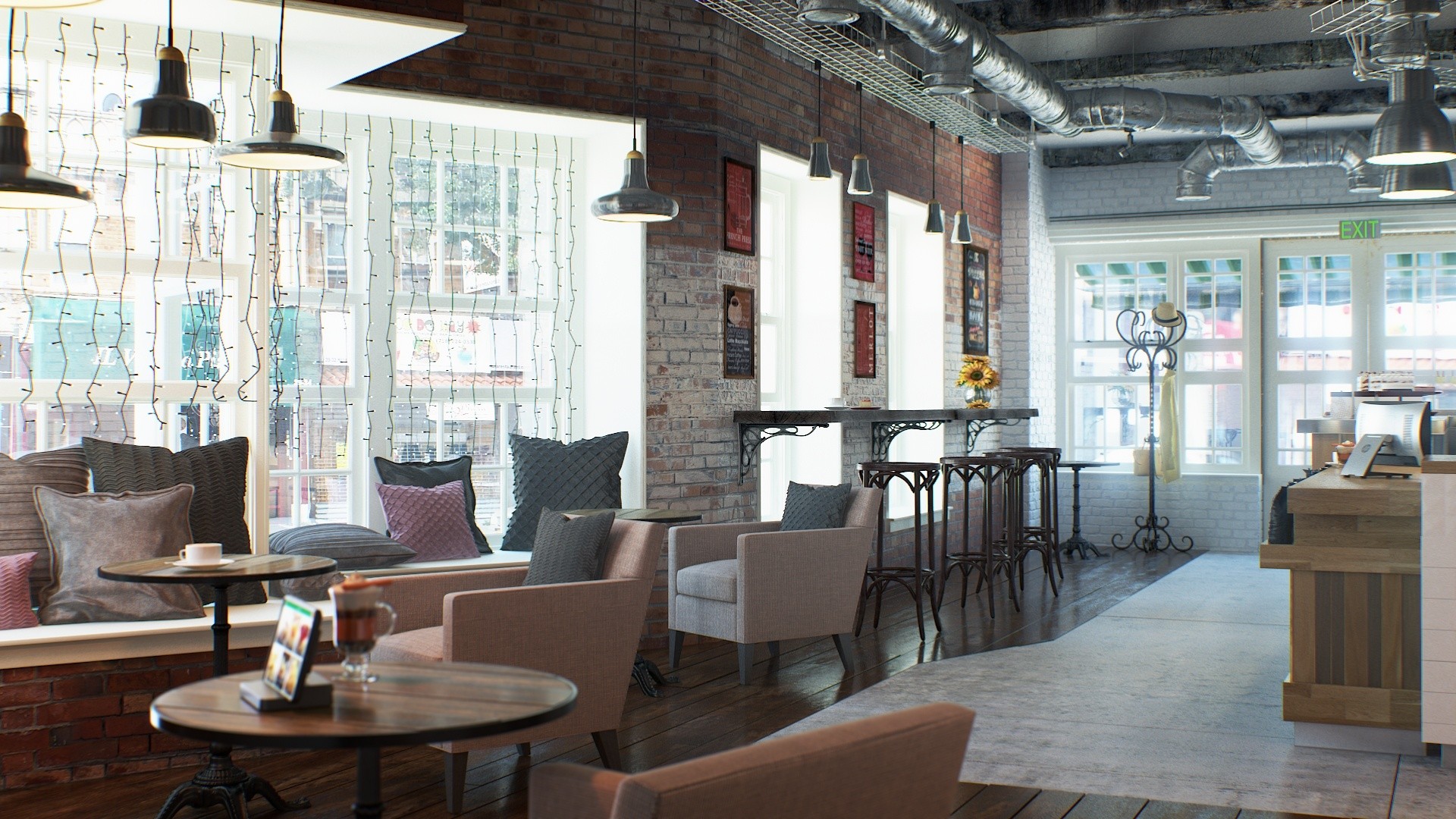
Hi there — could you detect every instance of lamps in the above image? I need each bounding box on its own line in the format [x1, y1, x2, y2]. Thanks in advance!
[925, 120, 944, 232]
[847, 80, 873, 196]
[0, 7, 93, 210]
[1365, 69, 1456, 166]
[592, 0, 681, 221]
[122, 0, 215, 149]
[219, 0, 347, 171]
[806, 60, 832, 179]
[949, 136, 972, 244]
[1378, 162, 1456, 200]
[1118, 132, 1138, 158]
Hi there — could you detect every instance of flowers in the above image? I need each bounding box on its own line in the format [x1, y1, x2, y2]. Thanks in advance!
[955, 353, 1002, 408]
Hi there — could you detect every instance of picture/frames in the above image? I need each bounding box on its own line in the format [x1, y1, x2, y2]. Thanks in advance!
[962, 243, 988, 356]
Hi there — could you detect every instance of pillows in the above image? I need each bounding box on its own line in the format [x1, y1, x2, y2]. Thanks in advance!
[0, 437, 492, 630]
[778, 481, 851, 532]
[501, 432, 630, 587]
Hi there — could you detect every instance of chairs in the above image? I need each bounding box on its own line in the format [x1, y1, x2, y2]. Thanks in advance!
[367, 486, 973, 819]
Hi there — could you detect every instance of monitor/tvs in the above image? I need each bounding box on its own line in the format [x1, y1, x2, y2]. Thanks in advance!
[1340, 400, 1432, 477]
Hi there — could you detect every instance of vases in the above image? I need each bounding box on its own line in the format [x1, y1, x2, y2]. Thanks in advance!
[963, 386, 992, 405]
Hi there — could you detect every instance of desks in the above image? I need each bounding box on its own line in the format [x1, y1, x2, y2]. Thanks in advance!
[98, 548, 343, 819]
[148, 659, 578, 819]
[733, 409, 1039, 485]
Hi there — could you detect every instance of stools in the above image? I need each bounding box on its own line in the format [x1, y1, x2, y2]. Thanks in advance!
[855, 444, 1061, 641]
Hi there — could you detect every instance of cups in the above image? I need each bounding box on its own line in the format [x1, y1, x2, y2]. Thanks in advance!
[831, 398, 846, 406]
[179, 543, 222, 564]
[328, 584, 396, 683]
[1335, 445, 1355, 466]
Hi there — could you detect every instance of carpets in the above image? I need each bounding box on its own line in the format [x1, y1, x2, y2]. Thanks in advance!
[754, 550, 1456, 819]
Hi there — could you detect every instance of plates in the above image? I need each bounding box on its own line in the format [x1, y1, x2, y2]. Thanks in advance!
[824, 407, 849, 410]
[1323, 462, 1342, 467]
[850, 406, 882, 410]
[173, 558, 234, 569]
